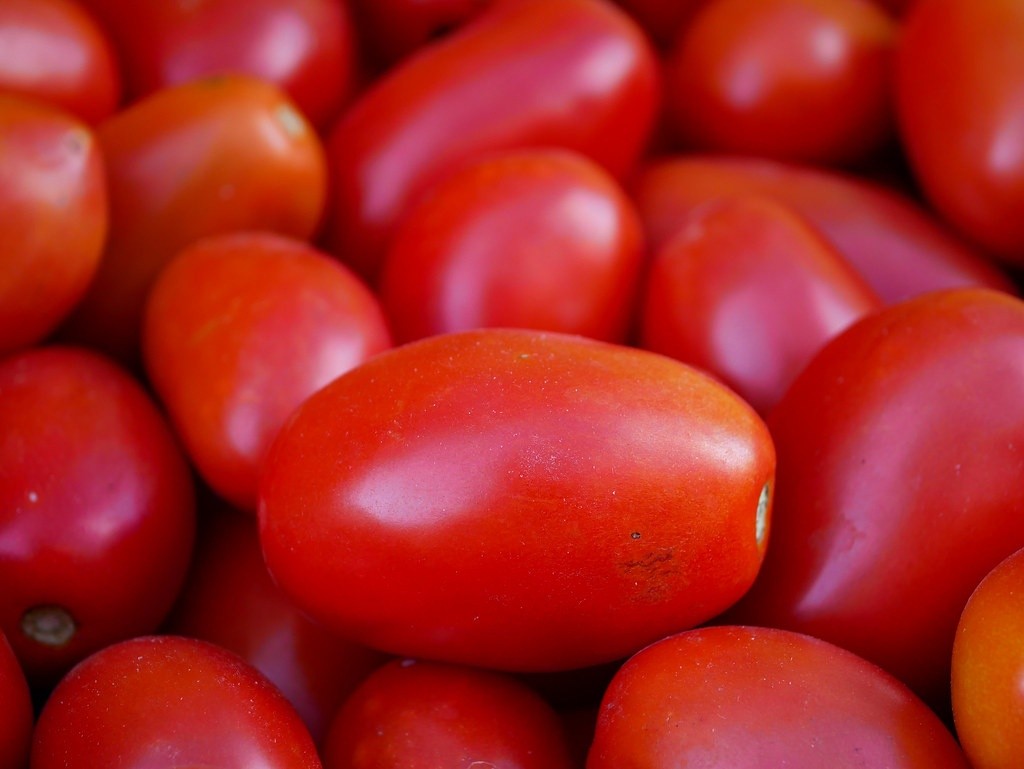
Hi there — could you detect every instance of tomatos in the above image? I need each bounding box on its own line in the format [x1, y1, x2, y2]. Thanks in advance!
[0, 1, 1024, 769]
